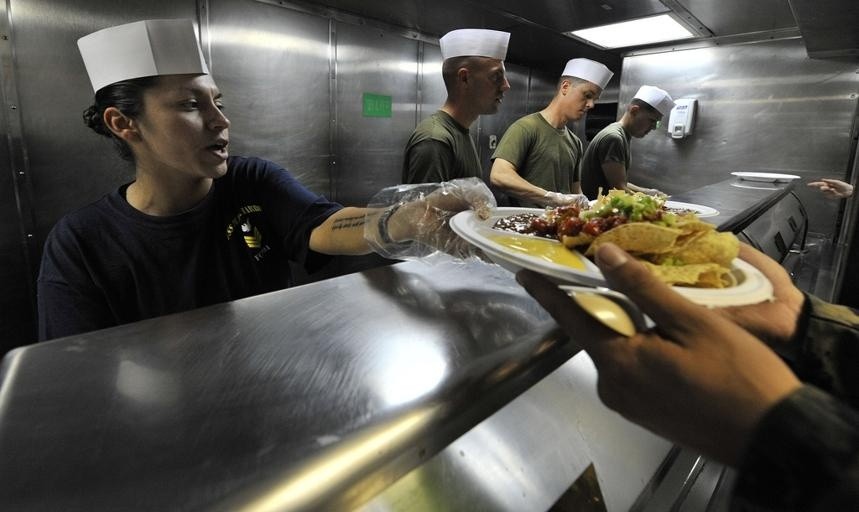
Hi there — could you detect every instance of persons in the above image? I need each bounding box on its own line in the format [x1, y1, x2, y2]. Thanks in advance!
[35, 17, 492, 344]
[489, 58, 614, 208]
[807, 177, 859, 201]
[580, 85, 675, 201]
[403, 28, 512, 253]
[514, 229, 859, 512]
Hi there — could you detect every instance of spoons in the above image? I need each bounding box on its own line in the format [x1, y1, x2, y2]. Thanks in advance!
[557, 282, 649, 337]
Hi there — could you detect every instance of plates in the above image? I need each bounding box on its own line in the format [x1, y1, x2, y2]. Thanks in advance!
[731, 171, 801, 188]
[448, 206, 775, 309]
[659, 201, 718, 219]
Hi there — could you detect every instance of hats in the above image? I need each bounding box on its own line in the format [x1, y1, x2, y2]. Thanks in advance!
[561, 58, 615, 90]
[438, 27, 512, 60]
[633, 85, 674, 116]
[77, 18, 210, 95]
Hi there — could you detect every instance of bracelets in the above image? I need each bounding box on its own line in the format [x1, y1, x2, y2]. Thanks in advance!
[377, 204, 414, 252]
